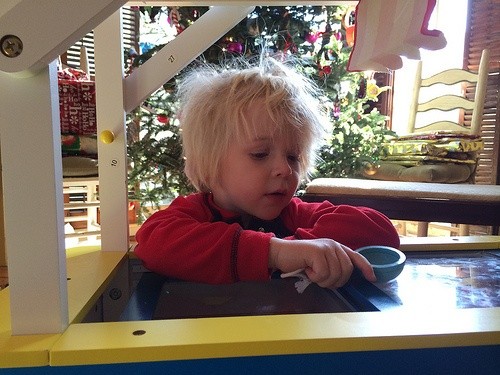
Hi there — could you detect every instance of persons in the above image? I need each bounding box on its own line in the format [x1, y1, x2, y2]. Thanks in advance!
[133, 39, 400, 289]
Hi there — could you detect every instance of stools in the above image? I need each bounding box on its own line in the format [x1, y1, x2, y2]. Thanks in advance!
[62, 155, 101, 238]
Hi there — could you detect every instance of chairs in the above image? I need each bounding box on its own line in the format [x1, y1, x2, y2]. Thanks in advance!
[361, 48, 489, 237]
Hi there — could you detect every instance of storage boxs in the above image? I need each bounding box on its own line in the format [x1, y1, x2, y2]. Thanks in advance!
[58, 78, 97, 135]
